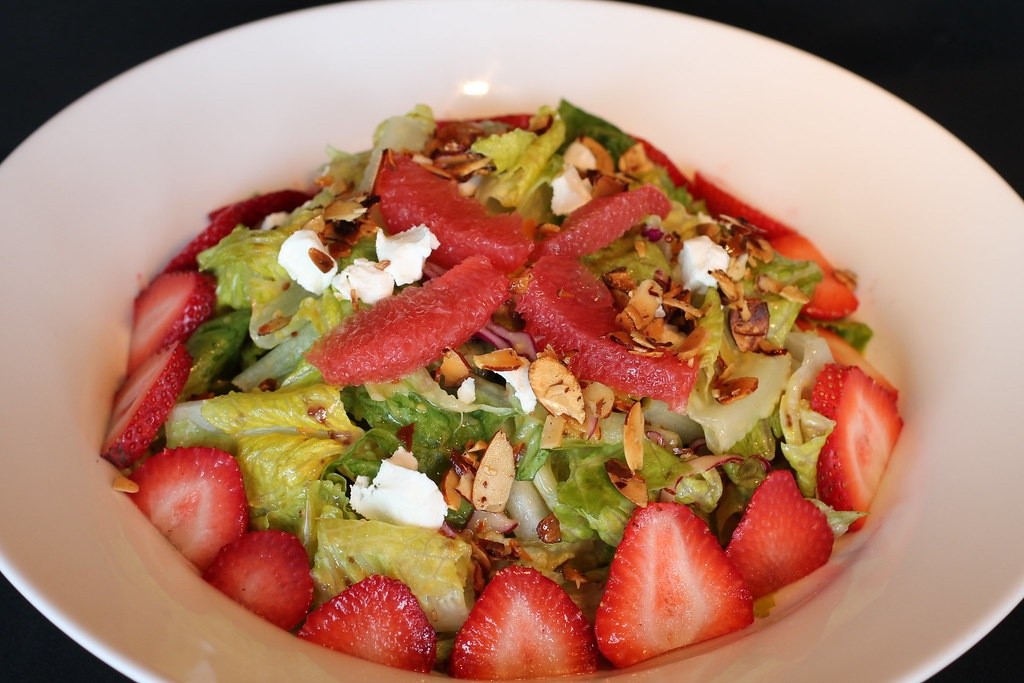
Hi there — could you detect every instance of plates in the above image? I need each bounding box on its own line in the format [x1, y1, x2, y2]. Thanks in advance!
[0, 0, 1024, 683]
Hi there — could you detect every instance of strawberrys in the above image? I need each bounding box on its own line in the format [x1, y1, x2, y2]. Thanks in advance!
[99, 171, 902, 683]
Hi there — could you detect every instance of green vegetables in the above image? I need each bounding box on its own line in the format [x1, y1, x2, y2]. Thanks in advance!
[165, 98, 872, 658]
